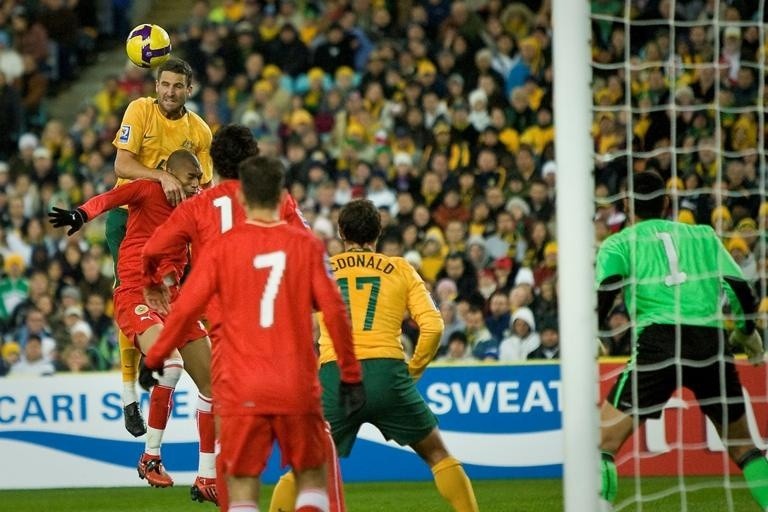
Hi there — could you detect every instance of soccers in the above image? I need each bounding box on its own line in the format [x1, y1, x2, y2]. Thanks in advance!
[127, 23, 172, 69]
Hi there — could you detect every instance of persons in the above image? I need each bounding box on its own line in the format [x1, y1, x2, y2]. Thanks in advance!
[137, 123, 345, 511]
[103, 57, 214, 439]
[47, 147, 222, 506]
[0, 0, 768, 367]
[268, 196, 480, 511]
[136, 155, 367, 511]
[594, 170, 768, 511]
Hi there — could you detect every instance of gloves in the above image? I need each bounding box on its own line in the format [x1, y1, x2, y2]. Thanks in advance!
[340, 381, 366, 417]
[729, 328, 765, 368]
[48, 207, 87, 235]
[139, 363, 163, 392]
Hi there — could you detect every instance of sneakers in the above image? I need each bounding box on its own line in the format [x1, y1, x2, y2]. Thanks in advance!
[190, 477, 220, 506]
[124, 402, 147, 437]
[138, 454, 174, 488]
